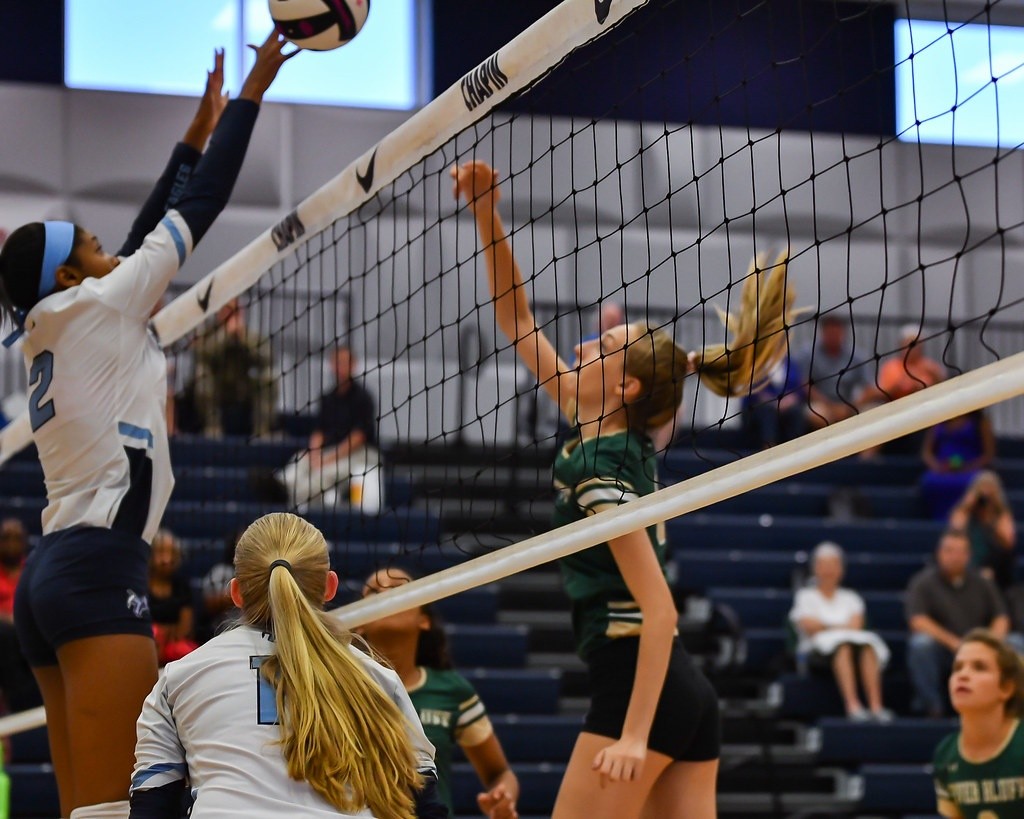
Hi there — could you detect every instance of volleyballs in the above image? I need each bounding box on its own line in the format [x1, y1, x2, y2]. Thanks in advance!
[267, 0, 372, 53]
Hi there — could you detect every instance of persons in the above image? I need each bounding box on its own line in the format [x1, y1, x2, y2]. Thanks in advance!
[0, 25, 1024, 819]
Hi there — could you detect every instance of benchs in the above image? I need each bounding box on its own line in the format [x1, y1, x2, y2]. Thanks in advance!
[0, 407, 1024, 819]
